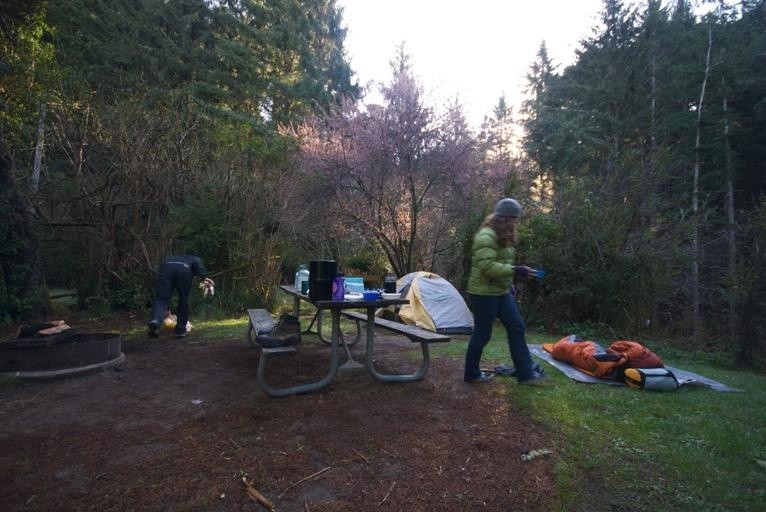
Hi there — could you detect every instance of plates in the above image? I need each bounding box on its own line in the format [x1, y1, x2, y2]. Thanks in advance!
[380, 293, 402, 300]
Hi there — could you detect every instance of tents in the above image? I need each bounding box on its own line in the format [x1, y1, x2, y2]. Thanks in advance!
[375, 270, 476, 334]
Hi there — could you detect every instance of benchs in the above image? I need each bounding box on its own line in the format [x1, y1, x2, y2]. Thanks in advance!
[246, 308, 451, 396]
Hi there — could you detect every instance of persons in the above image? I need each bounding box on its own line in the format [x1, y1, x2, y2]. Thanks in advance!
[464, 197, 553, 384]
[147, 255, 214, 338]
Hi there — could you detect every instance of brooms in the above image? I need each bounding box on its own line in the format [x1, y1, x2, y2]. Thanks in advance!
[338, 329, 367, 372]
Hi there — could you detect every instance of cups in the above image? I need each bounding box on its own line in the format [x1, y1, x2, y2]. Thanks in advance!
[384, 281, 396, 293]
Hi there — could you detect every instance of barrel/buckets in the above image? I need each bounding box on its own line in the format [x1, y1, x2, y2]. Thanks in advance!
[309, 259, 337, 301]
[297, 265, 309, 295]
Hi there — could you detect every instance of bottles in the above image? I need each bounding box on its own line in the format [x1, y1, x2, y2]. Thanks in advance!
[333, 272, 345, 301]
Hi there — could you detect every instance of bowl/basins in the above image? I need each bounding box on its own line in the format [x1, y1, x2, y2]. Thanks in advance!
[534, 270, 544, 278]
[362, 293, 380, 300]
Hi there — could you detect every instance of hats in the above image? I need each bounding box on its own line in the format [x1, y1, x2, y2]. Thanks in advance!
[494, 197, 522, 217]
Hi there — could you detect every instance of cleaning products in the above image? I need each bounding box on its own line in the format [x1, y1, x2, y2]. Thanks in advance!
[331, 272, 344, 301]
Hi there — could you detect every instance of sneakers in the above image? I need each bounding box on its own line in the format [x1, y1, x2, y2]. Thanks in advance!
[148, 320, 188, 337]
[464, 371, 560, 385]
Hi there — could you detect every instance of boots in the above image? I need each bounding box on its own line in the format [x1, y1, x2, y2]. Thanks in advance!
[255, 314, 302, 346]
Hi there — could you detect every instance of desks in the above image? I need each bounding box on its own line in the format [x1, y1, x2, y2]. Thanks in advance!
[279, 284, 410, 395]
